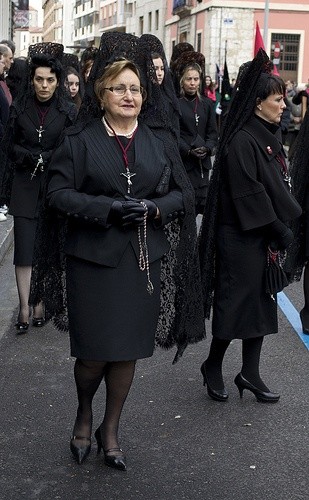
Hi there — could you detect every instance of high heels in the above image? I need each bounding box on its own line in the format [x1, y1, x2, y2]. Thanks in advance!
[234, 372, 280, 403]
[300, 308, 309, 335]
[200, 359, 229, 400]
[33, 305, 46, 326]
[16, 309, 31, 332]
[70, 414, 93, 466]
[95, 422, 127, 470]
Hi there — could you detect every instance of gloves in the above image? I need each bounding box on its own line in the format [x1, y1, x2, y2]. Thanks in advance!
[272, 228, 294, 249]
[25, 150, 50, 172]
[190, 146, 208, 160]
[108, 194, 157, 228]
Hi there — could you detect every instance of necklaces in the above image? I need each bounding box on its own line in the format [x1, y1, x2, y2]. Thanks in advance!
[182, 95, 200, 126]
[275, 154, 292, 194]
[33, 97, 51, 143]
[102, 115, 141, 194]
[101, 116, 137, 137]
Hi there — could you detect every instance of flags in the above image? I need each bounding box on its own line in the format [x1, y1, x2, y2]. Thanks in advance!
[254, 22, 280, 77]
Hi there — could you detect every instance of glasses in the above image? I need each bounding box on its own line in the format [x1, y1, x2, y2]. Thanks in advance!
[102, 85, 144, 96]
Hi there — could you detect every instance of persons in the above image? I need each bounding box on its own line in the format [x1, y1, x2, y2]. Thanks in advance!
[140, 50, 181, 149]
[0, 39, 26, 222]
[280, 112, 309, 334]
[198, 71, 301, 405]
[170, 61, 218, 220]
[59, 61, 309, 112]
[0, 54, 77, 331]
[24, 29, 205, 473]
